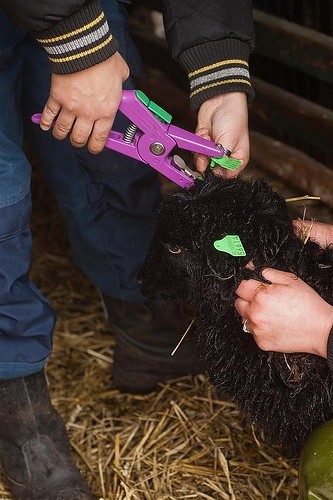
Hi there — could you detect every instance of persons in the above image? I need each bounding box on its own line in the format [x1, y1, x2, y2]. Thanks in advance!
[235, 220, 333, 500]
[1, 0, 252, 500]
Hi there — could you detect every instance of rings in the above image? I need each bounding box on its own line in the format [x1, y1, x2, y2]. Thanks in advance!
[241, 318, 250, 334]
[224, 148, 231, 157]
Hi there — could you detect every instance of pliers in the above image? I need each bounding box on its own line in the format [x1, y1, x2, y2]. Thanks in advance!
[29, 89, 225, 190]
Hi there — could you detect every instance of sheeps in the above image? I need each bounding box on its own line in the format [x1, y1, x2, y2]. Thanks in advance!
[137, 159, 333, 469]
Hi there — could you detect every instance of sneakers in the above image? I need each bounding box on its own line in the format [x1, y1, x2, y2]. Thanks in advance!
[100, 290, 204, 392]
[0, 369, 102, 500]
